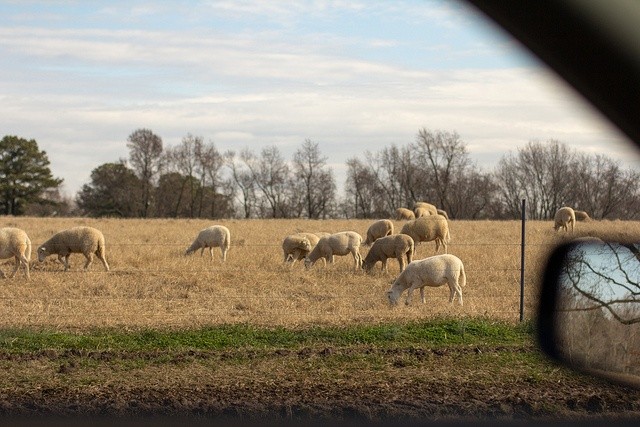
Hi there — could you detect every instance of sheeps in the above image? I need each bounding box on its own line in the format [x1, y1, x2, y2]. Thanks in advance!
[384, 254, 467, 307]
[399, 215, 449, 256]
[303, 231, 363, 275]
[360, 220, 394, 249]
[551, 206, 577, 235]
[37, 226, 110, 274]
[0, 226, 31, 280]
[395, 208, 416, 221]
[184, 225, 231, 262]
[361, 234, 415, 276]
[415, 202, 447, 216]
[282, 232, 327, 270]
